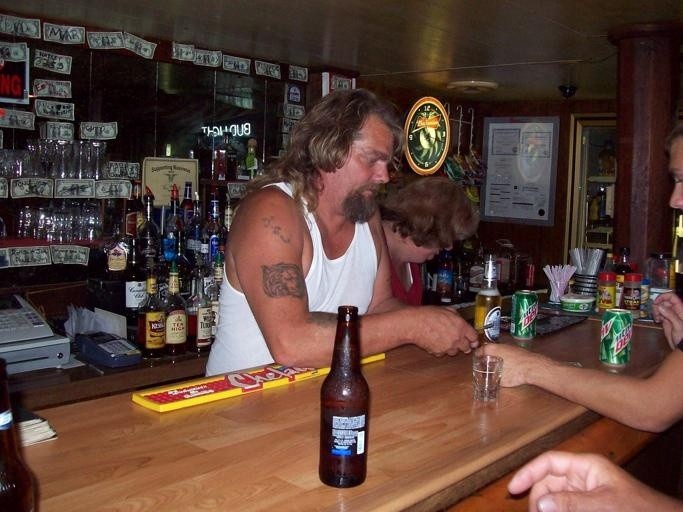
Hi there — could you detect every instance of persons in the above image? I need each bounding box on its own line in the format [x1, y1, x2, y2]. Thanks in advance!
[470, 117, 682, 438]
[201, 87, 480, 380]
[370, 176, 485, 308]
[505, 449, 682, 512]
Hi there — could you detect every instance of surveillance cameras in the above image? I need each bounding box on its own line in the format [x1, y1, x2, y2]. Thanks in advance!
[558, 82, 577, 98]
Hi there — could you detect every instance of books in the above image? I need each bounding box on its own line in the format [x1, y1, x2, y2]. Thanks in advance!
[10, 406, 58, 450]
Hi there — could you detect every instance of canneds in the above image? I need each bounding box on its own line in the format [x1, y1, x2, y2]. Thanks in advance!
[599, 308, 633, 368]
[511, 289, 539, 341]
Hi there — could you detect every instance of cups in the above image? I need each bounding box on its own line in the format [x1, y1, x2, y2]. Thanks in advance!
[0, 138, 103, 245]
[465, 402, 501, 449]
[472, 353, 505, 400]
[548, 281, 568, 305]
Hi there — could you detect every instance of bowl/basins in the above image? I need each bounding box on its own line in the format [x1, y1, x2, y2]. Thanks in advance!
[559, 295, 596, 314]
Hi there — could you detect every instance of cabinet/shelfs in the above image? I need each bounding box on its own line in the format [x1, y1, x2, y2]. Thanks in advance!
[0, 177, 249, 317]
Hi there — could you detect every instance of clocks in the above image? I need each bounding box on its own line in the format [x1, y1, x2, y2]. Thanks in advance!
[403, 96, 451, 177]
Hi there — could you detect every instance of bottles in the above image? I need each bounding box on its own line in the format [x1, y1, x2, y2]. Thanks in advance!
[0, 356, 41, 511]
[317, 304, 368, 489]
[423, 238, 484, 302]
[106, 138, 265, 359]
[472, 253, 501, 347]
[587, 140, 614, 228]
[594, 246, 674, 322]
